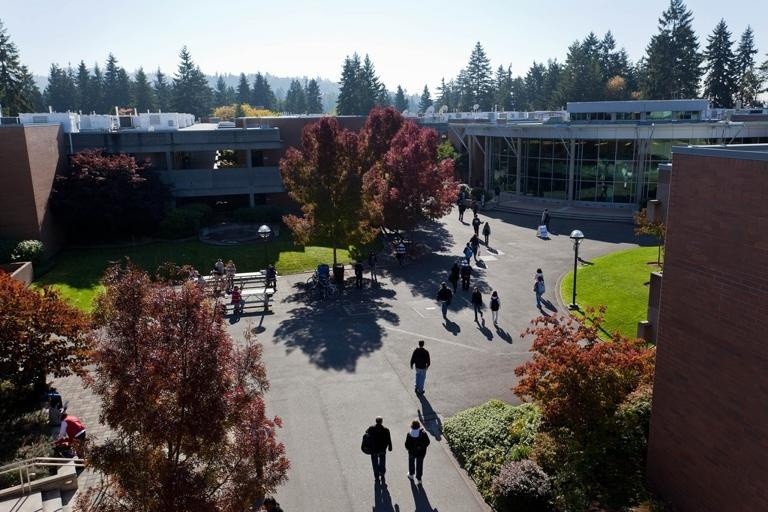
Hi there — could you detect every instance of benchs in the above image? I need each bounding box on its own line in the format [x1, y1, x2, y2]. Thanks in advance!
[204, 274, 277, 312]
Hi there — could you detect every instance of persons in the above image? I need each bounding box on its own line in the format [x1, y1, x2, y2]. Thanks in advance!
[599, 182, 608, 200]
[534, 276, 544, 308]
[405, 421, 430, 484]
[437, 187, 502, 325]
[355, 263, 364, 290]
[411, 340, 430, 394]
[264, 264, 278, 293]
[395, 240, 406, 266]
[365, 416, 392, 489]
[535, 268, 543, 283]
[215, 258, 245, 314]
[58, 414, 86, 464]
[541, 208, 551, 232]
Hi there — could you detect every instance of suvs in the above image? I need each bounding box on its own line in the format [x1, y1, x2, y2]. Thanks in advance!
[622, 158, 670, 192]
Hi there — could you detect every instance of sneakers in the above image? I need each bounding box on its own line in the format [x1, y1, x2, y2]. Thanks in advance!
[414, 388, 425, 393]
[406, 472, 414, 479]
[417, 478, 422, 483]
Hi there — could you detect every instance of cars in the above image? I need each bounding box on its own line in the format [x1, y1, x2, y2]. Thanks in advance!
[494, 158, 512, 186]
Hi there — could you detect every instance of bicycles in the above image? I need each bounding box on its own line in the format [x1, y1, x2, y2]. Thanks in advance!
[307, 269, 342, 299]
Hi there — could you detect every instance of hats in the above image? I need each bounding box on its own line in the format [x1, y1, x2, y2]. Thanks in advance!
[462, 260, 468, 263]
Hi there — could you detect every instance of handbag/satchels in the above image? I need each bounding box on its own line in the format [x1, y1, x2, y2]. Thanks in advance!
[361, 432, 371, 453]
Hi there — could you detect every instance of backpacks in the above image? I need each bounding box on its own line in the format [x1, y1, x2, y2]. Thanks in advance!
[491, 298, 499, 311]
[539, 282, 545, 293]
[466, 247, 473, 258]
[544, 214, 550, 223]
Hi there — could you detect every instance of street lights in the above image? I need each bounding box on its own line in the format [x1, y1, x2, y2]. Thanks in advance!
[565, 228, 586, 310]
[258, 223, 278, 294]
